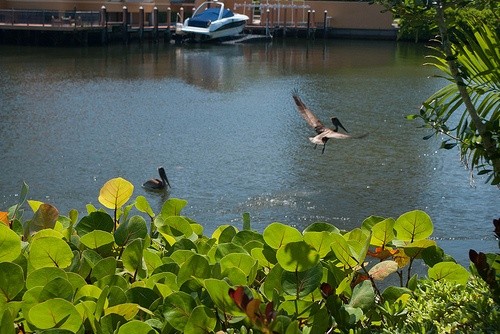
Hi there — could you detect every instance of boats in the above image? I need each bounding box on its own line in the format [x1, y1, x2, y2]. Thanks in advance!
[172, 1, 250, 43]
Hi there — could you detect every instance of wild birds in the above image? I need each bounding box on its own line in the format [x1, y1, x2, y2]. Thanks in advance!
[289, 88, 369, 155]
[141, 166, 171, 192]
